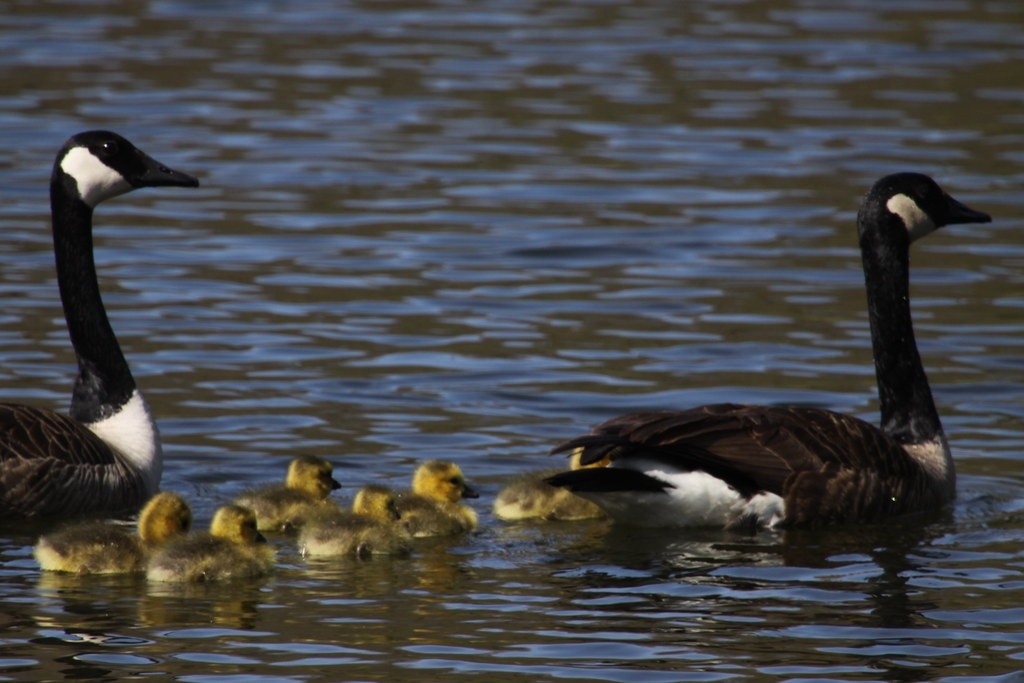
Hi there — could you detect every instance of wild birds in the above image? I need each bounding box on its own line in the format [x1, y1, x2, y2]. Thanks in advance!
[0, 130, 200, 536]
[147, 504, 278, 582]
[34, 493, 191, 578]
[392, 458, 480, 539]
[541, 171, 993, 542]
[235, 459, 342, 529]
[299, 485, 414, 560]
[496, 448, 614, 523]
